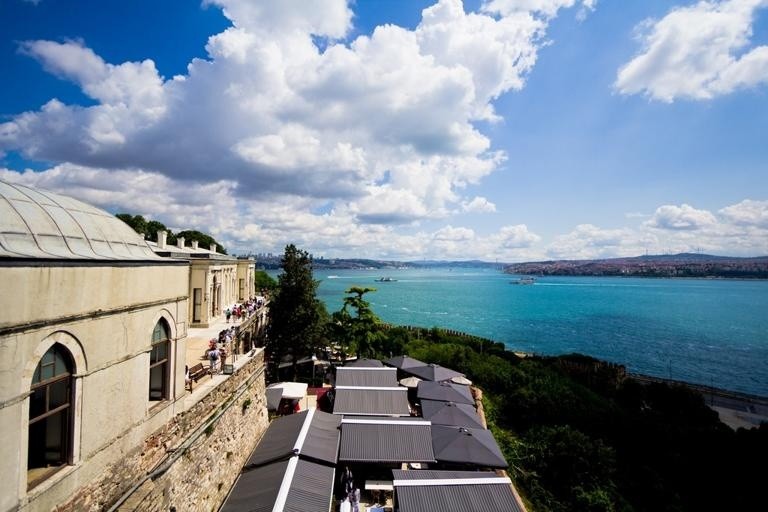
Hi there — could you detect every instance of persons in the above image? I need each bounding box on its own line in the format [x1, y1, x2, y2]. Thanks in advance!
[207, 347, 222, 372]
[217, 294, 263, 345]
[348, 487, 362, 512]
[341, 466, 354, 493]
[185, 364, 193, 391]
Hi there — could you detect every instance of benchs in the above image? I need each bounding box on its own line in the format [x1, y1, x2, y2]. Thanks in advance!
[188, 363, 212, 383]
